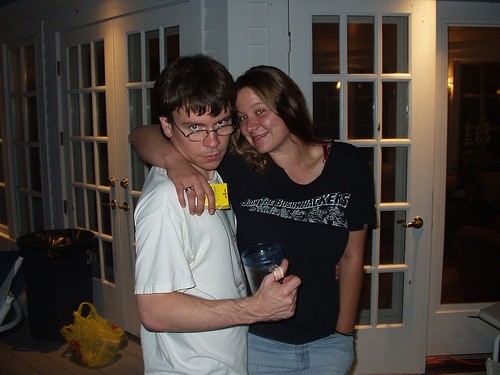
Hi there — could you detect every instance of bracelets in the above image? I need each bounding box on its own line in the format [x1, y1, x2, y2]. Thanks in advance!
[335, 329, 356, 336]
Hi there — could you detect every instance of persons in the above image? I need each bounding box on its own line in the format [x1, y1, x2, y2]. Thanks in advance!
[133, 54, 302, 375]
[127, 65, 375, 375]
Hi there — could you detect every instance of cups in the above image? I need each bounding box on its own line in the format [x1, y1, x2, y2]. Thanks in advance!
[242, 241, 285, 297]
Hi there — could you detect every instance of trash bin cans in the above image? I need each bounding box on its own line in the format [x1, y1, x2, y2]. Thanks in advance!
[16, 229, 95, 341]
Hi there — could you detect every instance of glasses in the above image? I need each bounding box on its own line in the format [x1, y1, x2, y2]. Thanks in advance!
[166, 113, 240, 142]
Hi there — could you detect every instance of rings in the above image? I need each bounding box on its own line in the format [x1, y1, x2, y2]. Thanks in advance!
[184, 187, 194, 192]
